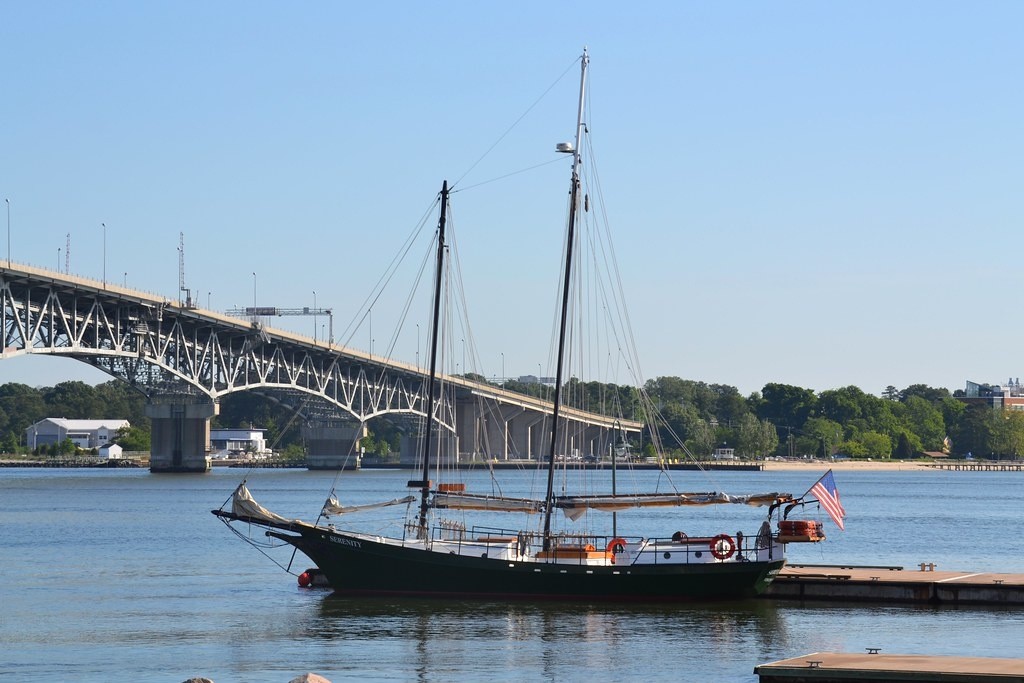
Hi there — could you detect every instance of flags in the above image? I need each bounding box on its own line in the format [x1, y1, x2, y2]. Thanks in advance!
[809, 469, 846, 532]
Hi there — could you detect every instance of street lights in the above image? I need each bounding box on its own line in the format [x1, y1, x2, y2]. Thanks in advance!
[6, 198, 11, 270]
[125, 273, 127, 288]
[416, 324, 420, 370]
[501, 353, 505, 391]
[102, 223, 106, 290]
[461, 339, 466, 382]
[368, 309, 372, 356]
[539, 364, 542, 402]
[58, 248, 60, 273]
[253, 272, 257, 322]
[312, 290, 316, 340]
[207, 292, 211, 311]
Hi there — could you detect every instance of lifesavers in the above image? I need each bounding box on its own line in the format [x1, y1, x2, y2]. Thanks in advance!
[605, 538, 630, 564]
[710, 534, 735, 559]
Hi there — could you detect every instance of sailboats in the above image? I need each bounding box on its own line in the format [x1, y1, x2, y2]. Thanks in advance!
[211, 43, 827, 603]
